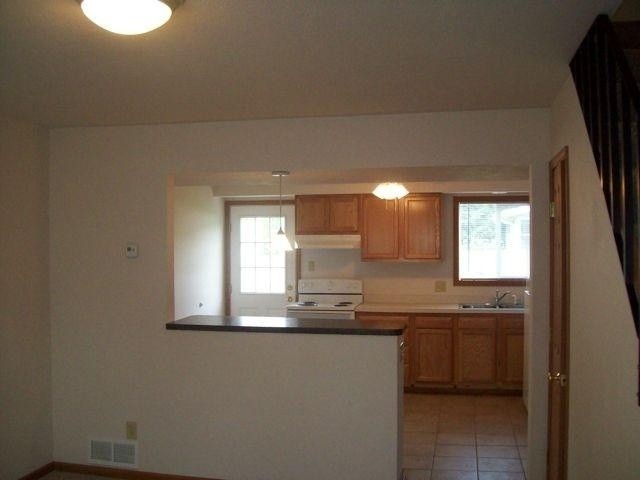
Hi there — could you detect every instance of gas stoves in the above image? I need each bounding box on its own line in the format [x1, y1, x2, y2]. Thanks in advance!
[284, 294, 363, 312]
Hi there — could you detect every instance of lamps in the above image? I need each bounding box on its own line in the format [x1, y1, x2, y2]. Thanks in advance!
[76, 0, 184, 35]
[271, 170, 294, 251]
[371, 180, 410, 200]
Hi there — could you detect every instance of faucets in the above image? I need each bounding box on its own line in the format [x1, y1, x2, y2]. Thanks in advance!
[495, 290, 511, 302]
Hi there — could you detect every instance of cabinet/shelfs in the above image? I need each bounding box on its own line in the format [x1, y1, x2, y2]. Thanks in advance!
[355, 312, 525, 397]
[361, 194, 441, 262]
[294, 193, 361, 234]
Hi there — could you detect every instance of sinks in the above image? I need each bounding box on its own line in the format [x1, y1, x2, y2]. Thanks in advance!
[457, 303, 497, 309]
[498, 303, 524, 310]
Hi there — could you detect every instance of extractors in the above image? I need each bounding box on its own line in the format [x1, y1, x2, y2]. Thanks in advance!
[296, 235, 361, 249]
